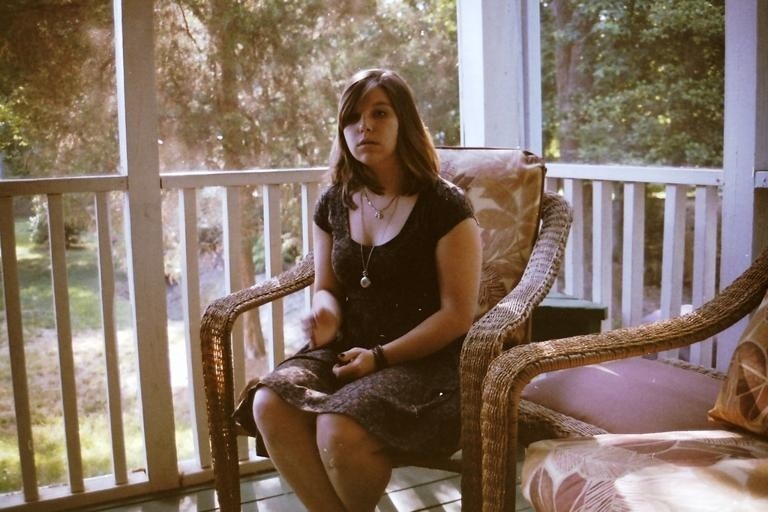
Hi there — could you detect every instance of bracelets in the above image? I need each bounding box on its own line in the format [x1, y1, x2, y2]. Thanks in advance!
[373, 343, 389, 371]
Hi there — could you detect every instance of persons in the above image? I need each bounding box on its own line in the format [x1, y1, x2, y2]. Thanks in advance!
[230, 65, 483, 512]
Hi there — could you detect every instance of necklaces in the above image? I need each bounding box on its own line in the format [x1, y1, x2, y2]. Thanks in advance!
[361, 184, 399, 218]
[358, 196, 399, 289]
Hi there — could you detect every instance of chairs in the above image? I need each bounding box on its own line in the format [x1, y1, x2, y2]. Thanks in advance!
[478, 246, 764, 507]
[200, 177, 574, 508]
